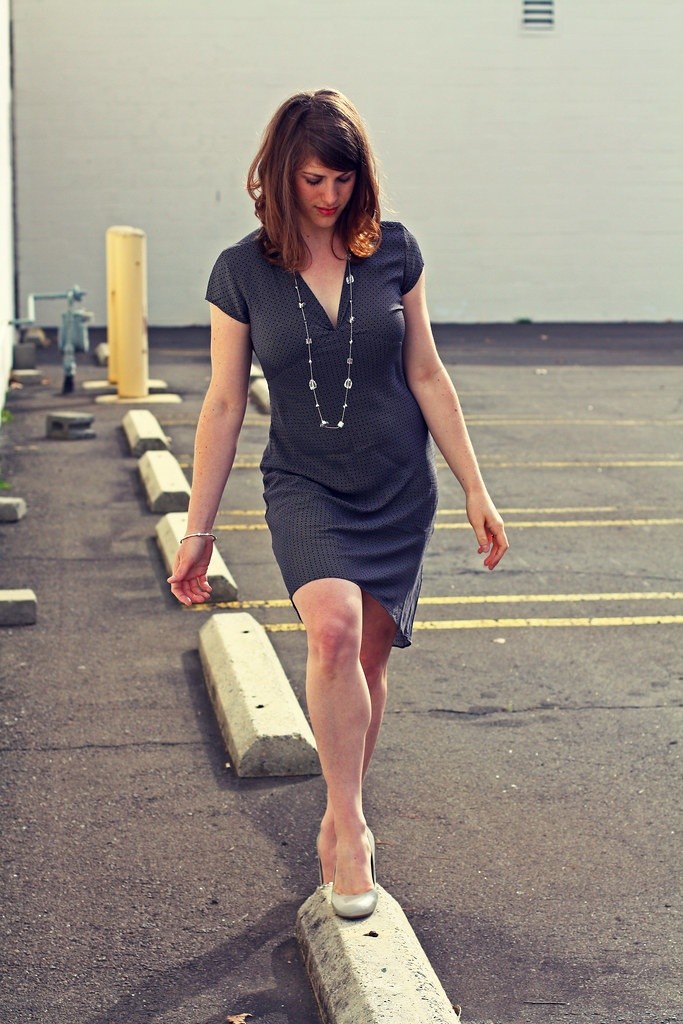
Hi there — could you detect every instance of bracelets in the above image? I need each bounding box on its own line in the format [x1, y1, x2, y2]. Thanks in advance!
[180, 533, 217, 544]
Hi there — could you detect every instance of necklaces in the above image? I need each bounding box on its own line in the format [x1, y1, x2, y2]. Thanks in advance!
[292, 253, 356, 430]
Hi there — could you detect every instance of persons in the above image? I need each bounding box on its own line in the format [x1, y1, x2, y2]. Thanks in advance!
[165, 87, 509, 918]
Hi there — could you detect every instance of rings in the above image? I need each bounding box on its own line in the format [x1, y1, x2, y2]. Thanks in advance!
[492, 535, 495, 538]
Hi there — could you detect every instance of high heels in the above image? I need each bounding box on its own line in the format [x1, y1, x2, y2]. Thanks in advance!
[331, 826, 376, 919]
[316, 833, 336, 886]
